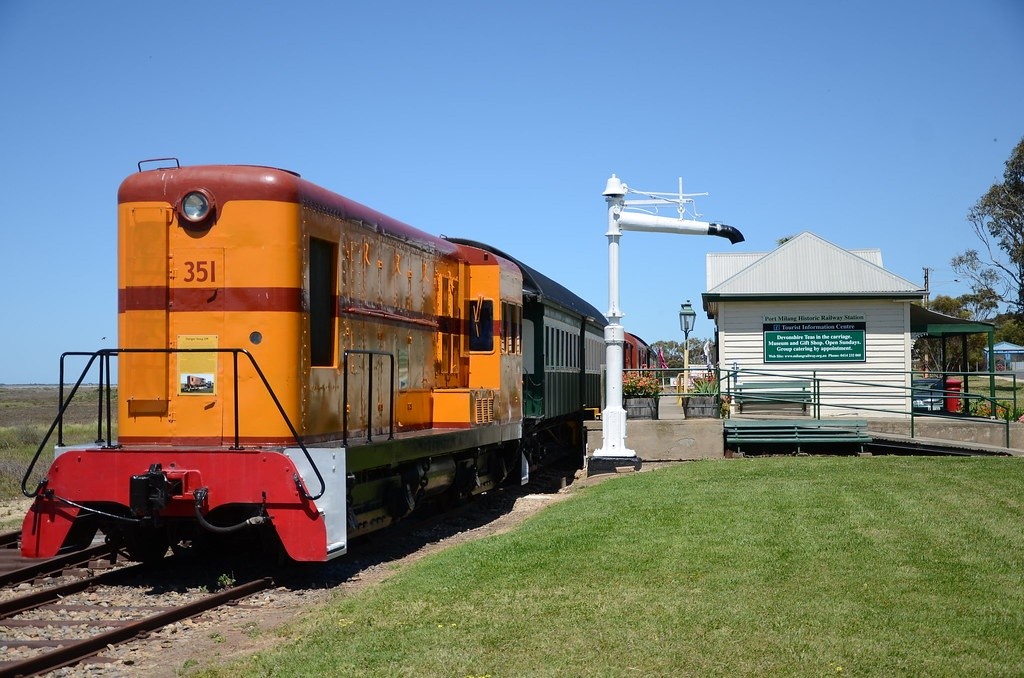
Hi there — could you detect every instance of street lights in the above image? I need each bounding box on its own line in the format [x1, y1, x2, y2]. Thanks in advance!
[680, 300, 696, 393]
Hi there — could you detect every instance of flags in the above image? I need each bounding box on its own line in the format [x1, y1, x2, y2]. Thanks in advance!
[657, 351, 669, 368]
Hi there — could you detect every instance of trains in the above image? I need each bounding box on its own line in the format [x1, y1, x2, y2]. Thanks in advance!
[20, 158, 657, 574]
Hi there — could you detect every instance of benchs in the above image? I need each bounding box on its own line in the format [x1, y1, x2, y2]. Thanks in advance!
[733, 379, 812, 415]
[723, 419, 872, 458]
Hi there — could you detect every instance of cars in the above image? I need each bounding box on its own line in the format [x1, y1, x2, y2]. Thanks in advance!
[913, 378, 943, 411]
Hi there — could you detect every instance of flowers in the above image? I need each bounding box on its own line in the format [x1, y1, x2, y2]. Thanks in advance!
[622, 371, 664, 398]
[682, 372, 730, 418]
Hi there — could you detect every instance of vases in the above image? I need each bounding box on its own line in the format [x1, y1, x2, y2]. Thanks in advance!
[622, 398, 659, 420]
[681, 397, 721, 419]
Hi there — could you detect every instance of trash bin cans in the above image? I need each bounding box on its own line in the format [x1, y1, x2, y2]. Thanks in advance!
[946, 378, 962, 413]
